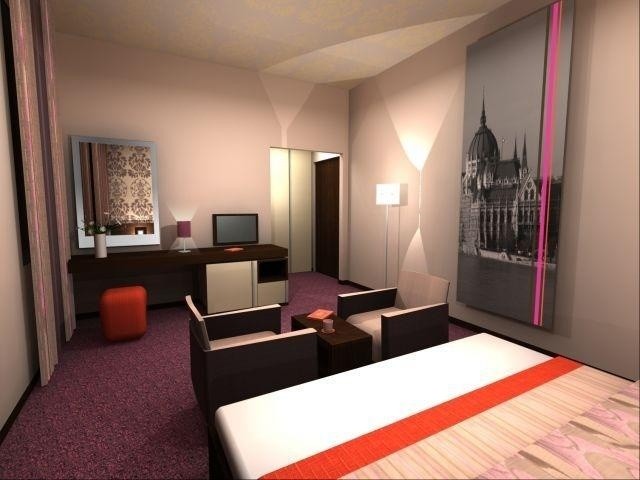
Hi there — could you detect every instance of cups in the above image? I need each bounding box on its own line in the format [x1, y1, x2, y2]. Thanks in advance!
[323, 319, 335, 332]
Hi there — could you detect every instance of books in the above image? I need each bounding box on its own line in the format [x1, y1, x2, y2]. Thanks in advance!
[307, 309, 333, 320]
[224, 248, 244, 252]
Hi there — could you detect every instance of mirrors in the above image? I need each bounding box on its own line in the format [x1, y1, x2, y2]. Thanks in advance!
[70, 132, 162, 248]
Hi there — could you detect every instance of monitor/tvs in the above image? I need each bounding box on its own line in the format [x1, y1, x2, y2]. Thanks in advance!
[212, 213, 258, 246]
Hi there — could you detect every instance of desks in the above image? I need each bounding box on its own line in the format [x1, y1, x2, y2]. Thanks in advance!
[65, 243, 292, 323]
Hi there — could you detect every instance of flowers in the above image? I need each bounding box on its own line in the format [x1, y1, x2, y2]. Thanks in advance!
[81, 219, 109, 235]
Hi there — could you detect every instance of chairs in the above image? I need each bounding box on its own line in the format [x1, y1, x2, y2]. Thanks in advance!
[337, 267, 456, 367]
[179, 289, 325, 427]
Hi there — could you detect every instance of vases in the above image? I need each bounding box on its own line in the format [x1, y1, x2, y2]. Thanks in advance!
[92, 234, 107, 259]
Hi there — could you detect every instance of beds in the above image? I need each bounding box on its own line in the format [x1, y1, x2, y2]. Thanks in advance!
[206, 329, 640, 480]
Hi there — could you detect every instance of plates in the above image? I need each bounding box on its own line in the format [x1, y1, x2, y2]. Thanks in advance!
[321, 329, 336, 334]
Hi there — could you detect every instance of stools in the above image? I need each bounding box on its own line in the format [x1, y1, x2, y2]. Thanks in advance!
[98, 285, 149, 344]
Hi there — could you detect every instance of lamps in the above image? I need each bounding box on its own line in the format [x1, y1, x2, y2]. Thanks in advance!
[374, 182, 402, 292]
[176, 220, 193, 253]
